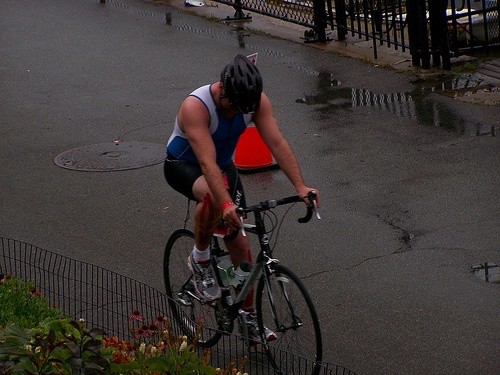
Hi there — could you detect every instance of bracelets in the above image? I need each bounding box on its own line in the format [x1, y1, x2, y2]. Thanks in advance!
[218, 202, 234, 213]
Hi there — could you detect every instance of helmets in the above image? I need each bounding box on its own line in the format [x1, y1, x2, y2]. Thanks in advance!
[222, 54, 263, 115]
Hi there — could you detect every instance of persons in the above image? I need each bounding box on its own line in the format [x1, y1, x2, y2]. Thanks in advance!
[163, 55, 321, 347]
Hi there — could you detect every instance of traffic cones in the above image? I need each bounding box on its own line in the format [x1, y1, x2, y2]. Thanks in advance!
[232, 121, 279, 175]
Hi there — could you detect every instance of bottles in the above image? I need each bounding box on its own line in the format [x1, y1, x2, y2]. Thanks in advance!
[219, 252, 236, 287]
[229, 261, 251, 288]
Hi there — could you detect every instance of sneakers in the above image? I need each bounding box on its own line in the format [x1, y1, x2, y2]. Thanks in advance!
[236, 309, 277, 344]
[186, 249, 222, 301]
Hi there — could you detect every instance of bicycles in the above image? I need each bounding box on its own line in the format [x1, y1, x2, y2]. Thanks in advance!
[162, 187, 324, 375]
